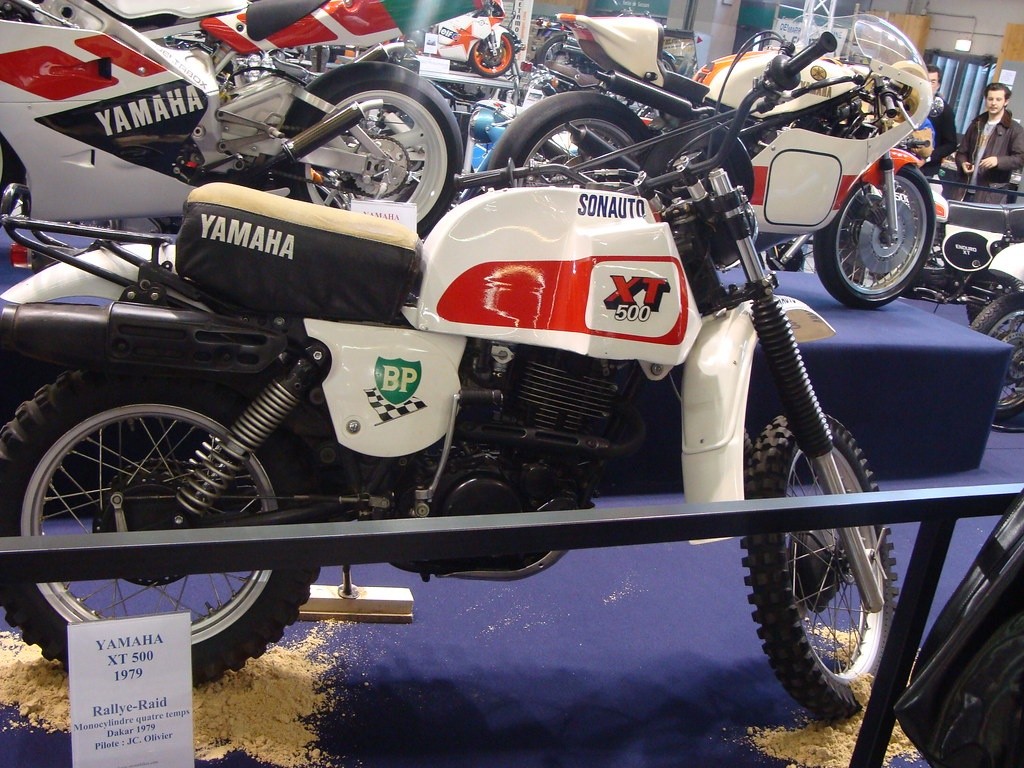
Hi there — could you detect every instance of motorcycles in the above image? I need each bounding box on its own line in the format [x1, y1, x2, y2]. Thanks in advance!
[2, 0, 1024, 721]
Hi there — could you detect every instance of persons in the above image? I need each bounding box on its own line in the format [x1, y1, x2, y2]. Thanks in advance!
[918, 65, 958, 181]
[955, 83, 1024, 203]
